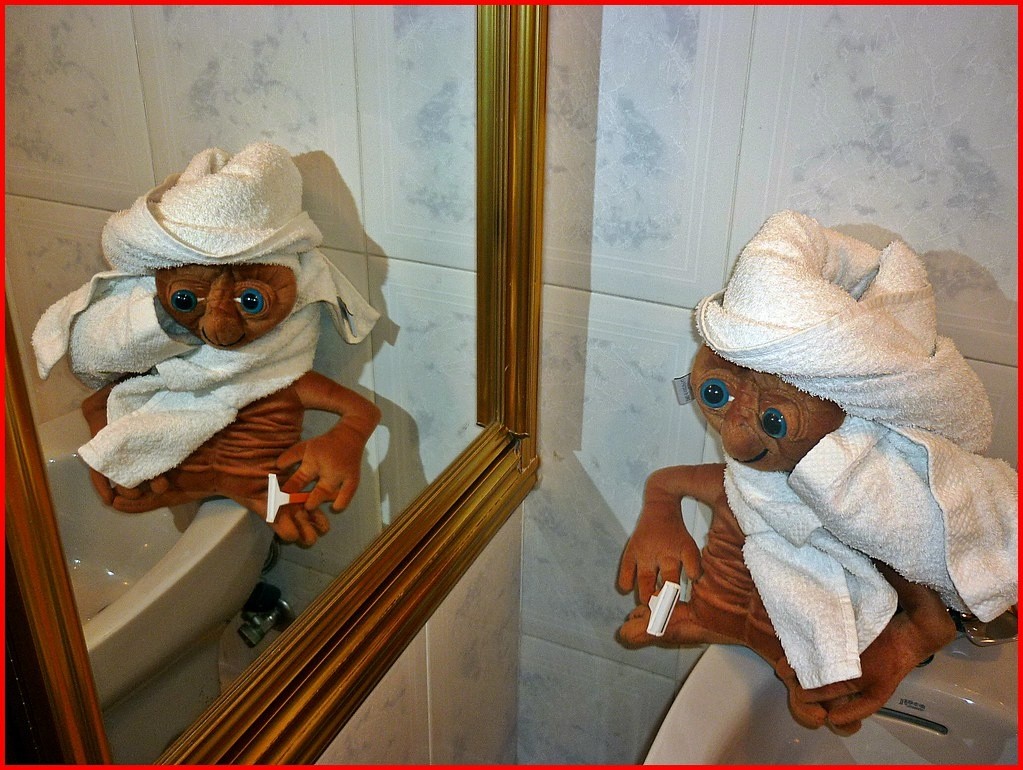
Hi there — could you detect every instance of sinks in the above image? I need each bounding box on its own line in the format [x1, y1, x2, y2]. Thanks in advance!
[30, 409, 273, 732]
[638, 600, 1022, 766]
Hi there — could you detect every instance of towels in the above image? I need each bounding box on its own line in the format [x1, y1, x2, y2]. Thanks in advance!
[28, 140, 383, 490]
[694, 208, 1021, 695]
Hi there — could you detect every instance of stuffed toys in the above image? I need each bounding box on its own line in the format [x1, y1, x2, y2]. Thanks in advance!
[613, 212, 1020, 735]
[31, 142, 384, 545]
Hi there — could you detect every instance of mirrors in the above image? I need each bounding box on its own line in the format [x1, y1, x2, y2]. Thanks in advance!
[5, 2, 555, 770]
